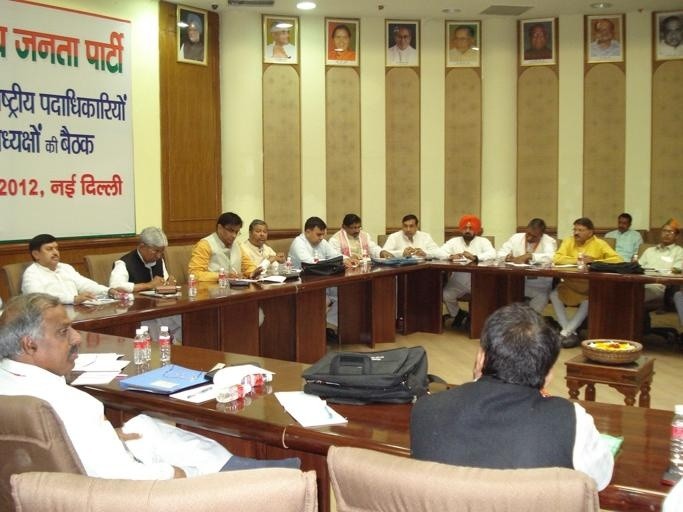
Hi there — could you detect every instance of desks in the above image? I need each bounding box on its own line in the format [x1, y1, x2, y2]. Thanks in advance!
[286, 381, 683, 512]
[564, 355, 657, 406]
[295, 254, 429, 361]
[430, 260, 683, 350]
[71, 328, 338, 460]
[62, 280, 296, 360]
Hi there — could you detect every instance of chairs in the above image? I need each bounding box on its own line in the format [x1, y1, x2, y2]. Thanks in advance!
[598, 237, 617, 250]
[326, 445, 597, 511]
[377, 234, 388, 246]
[8, 468, 319, 512]
[484, 235, 496, 246]
[638, 243, 655, 257]
[266, 238, 296, 258]
[160, 244, 193, 283]
[554, 238, 563, 248]
[2, 262, 30, 298]
[0, 393, 85, 479]
[648, 226, 661, 243]
[85, 251, 128, 284]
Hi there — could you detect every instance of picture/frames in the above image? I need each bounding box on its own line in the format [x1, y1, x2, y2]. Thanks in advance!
[584, 14, 627, 64]
[261, 13, 301, 65]
[444, 19, 482, 68]
[519, 18, 557, 66]
[175, 3, 209, 67]
[324, 17, 360, 67]
[651, 10, 683, 62]
[384, 18, 422, 68]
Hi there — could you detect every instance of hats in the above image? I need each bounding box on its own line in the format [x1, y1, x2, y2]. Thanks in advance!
[668, 218, 681, 234]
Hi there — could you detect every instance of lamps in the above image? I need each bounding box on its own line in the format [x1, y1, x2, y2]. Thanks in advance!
[442, 7, 461, 14]
[591, 1, 614, 10]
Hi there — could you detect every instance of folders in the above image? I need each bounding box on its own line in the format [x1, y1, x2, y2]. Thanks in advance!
[118, 364, 212, 395]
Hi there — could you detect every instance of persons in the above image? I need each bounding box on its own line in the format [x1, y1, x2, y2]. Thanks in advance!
[496, 218, 558, 314]
[382, 214, 440, 261]
[409, 303, 614, 494]
[267, 21, 296, 62]
[433, 215, 496, 329]
[286, 217, 360, 342]
[450, 25, 480, 67]
[187, 211, 264, 280]
[242, 219, 286, 279]
[327, 213, 395, 265]
[0, 291, 187, 481]
[20, 233, 129, 306]
[325, 25, 356, 63]
[548, 217, 625, 349]
[524, 21, 553, 60]
[657, 15, 683, 57]
[387, 23, 417, 66]
[107, 226, 179, 344]
[591, 18, 618, 57]
[638, 218, 683, 303]
[178, 14, 205, 64]
[603, 213, 644, 263]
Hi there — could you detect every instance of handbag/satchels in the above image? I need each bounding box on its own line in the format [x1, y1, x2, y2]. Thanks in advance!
[307, 345, 430, 405]
[302, 256, 344, 277]
[589, 261, 644, 275]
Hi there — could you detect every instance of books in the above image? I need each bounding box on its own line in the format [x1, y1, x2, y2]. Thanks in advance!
[118, 355, 277, 409]
[273, 390, 348, 431]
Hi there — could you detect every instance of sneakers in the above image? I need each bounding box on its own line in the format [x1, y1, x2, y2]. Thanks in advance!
[452, 310, 467, 329]
[562, 334, 579, 348]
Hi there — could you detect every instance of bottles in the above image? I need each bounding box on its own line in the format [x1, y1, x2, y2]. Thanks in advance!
[633, 255, 639, 263]
[188, 274, 195, 296]
[577, 253, 584, 269]
[363, 250, 367, 265]
[242, 373, 273, 387]
[141, 325, 151, 361]
[159, 326, 171, 364]
[314, 250, 319, 262]
[134, 329, 146, 365]
[668, 405, 683, 472]
[286, 257, 292, 271]
[215, 382, 251, 403]
[115, 293, 132, 299]
[399, 317, 404, 332]
[218, 268, 226, 289]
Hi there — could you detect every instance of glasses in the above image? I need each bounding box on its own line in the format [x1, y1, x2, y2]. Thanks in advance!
[146, 247, 166, 256]
[224, 225, 241, 236]
